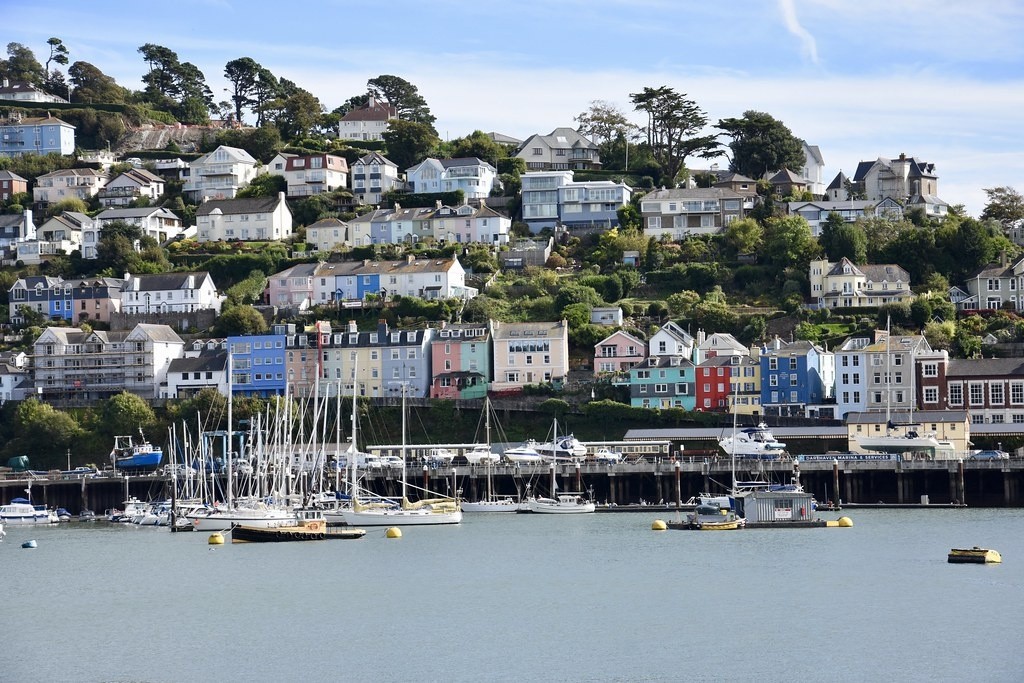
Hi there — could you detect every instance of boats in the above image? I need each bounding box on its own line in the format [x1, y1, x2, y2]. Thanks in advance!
[58, 511, 71, 522]
[0, 504, 51, 524]
[79, 510, 96, 522]
[110, 428, 163, 475]
[719, 428, 786, 460]
[682, 518, 746, 530]
[533, 432, 587, 457]
[503, 439, 542, 462]
[231, 510, 367, 543]
[464, 446, 501, 464]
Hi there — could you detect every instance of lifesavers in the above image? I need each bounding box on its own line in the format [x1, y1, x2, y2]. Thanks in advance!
[310, 523, 318, 531]
[301, 532, 308, 540]
[320, 533, 325, 539]
[294, 532, 301, 539]
[275, 530, 282, 538]
[310, 533, 317, 540]
[286, 532, 293, 540]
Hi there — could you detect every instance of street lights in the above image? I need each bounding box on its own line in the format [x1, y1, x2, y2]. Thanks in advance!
[67, 449, 70, 471]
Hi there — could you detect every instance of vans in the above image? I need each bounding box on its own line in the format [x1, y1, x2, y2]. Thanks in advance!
[368, 456, 404, 469]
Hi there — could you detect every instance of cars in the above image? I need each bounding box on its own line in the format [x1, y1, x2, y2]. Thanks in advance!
[969, 450, 1010, 461]
[585, 449, 618, 462]
[75, 467, 90, 471]
[324, 458, 351, 469]
[224, 460, 252, 475]
[421, 449, 455, 463]
[164, 464, 191, 475]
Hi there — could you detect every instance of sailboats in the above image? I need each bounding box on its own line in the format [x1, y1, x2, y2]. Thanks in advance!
[851, 316, 939, 452]
[129, 322, 462, 530]
[527, 417, 596, 513]
[463, 395, 519, 512]
